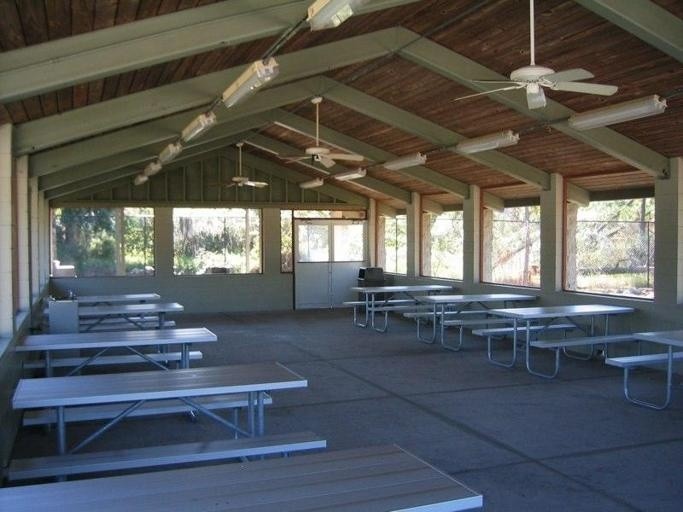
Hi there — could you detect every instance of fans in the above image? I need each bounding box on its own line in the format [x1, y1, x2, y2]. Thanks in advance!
[223, 141, 269, 187]
[278, 94, 364, 170]
[452, 0, 619, 111]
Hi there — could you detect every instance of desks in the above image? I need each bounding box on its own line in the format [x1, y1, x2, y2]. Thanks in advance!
[43, 292, 161, 304]
[472, 303, 638, 380]
[15, 326, 220, 376]
[343, 286, 453, 333]
[1, 441, 486, 512]
[403, 293, 538, 352]
[7, 359, 328, 486]
[42, 301, 186, 334]
[604, 329, 682, 411]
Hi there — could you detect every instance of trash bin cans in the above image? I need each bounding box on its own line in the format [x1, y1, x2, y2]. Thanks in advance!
[359, 267, 383, 309]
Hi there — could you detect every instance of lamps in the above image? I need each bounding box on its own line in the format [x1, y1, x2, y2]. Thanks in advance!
[182, 112, 216, 145]
[159, 144, 183, 164]
[453, 130, 520, 157]
[332, 169, 367, 183]
[306, 1, 355, 35]
[298, 178, 324, 190]
[566, 95, 666, 133]
[382, 152, 426, 173]
[223, 56, 279, 111]
[135, 163, 163, 188]
